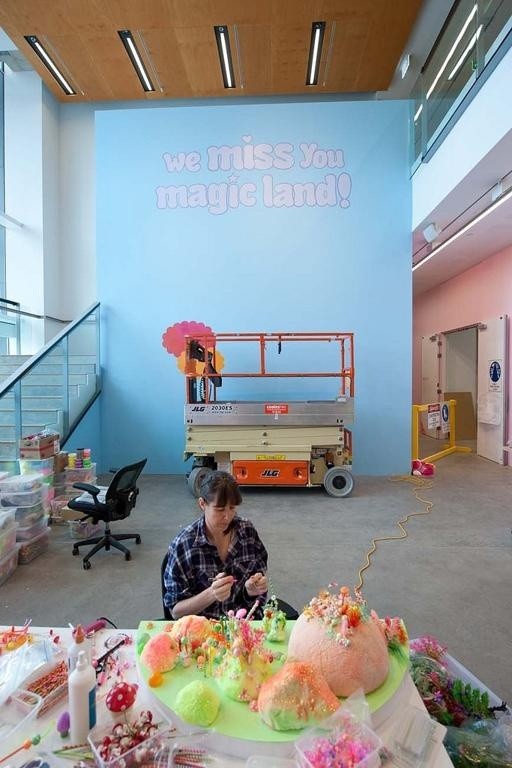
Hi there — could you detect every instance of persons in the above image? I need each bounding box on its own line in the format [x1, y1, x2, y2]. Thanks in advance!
[162, 470, 269, 621]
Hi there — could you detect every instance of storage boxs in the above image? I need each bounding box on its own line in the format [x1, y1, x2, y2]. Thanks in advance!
[0, 431, 108, 588]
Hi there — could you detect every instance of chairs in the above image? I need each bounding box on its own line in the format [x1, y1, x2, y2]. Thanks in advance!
[68, 458, 148, 569]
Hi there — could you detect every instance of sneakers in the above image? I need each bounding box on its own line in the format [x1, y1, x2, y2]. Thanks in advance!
[412, 459, 434, 476]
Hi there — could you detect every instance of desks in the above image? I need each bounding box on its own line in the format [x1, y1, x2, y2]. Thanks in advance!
[1, 619, 458, 768]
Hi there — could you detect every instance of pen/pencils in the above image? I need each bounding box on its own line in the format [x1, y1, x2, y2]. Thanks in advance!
[93, 636, 129, 668]
[206, 578, 239, 583]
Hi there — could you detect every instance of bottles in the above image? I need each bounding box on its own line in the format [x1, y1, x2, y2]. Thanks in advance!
[69, 648, 98, 745]
[69, 623, 92, 670]
